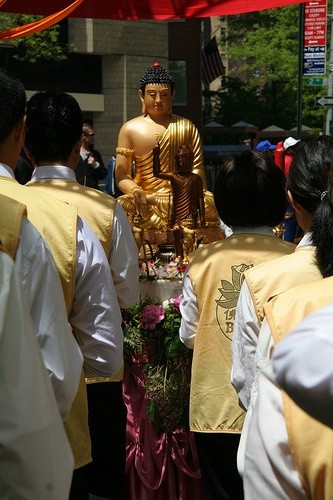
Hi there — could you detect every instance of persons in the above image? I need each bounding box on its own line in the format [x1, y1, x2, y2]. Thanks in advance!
[180, 135, 333, 500]
[153, 142, 207, 253]
[113, 60, 226, 258]
[0, 69, 139, 500]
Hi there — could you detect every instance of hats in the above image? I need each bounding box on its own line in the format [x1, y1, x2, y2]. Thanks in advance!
[255, 140, 276, 153]
[282, 137, 300, 150]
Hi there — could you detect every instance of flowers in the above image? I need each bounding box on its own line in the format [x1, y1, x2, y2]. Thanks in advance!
[142, 256, 190, 280]
[140, 294, 182, 330]
